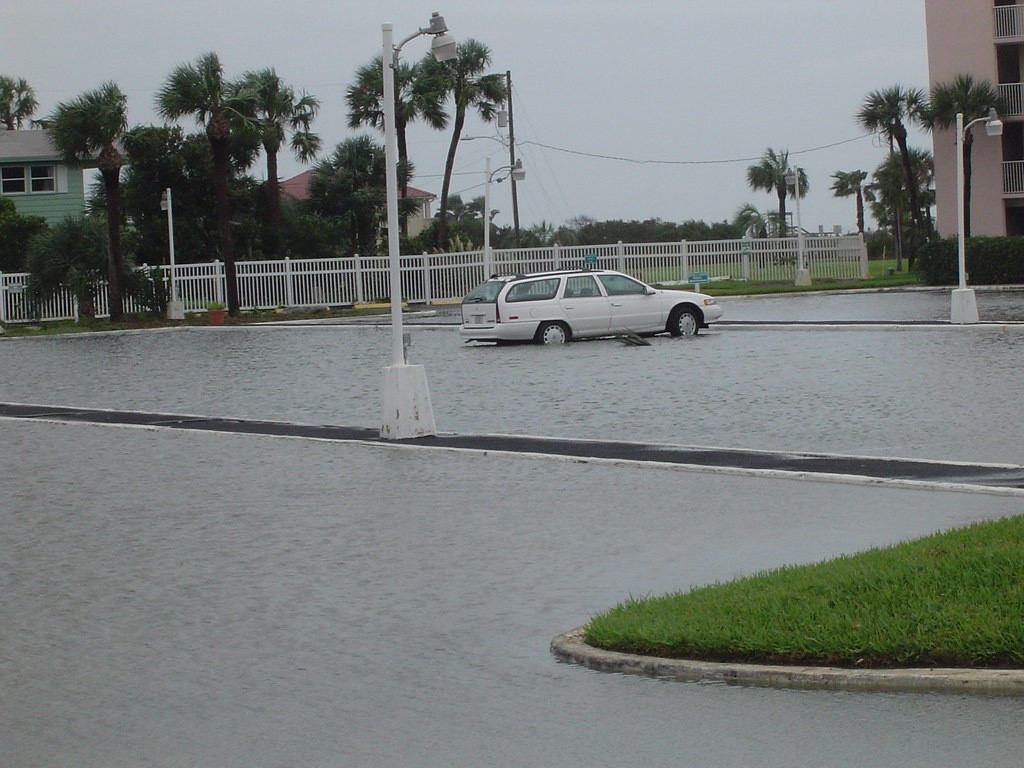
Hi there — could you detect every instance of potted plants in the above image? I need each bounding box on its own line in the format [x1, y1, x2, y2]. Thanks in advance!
[206, 301, 225, 325]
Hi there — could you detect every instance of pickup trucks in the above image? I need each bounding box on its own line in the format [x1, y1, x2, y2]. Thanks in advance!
[459, 267, 723, 345]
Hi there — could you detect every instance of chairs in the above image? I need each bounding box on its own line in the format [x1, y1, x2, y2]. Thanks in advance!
[580, 289, 594, 297]
[565, 289, 573, 297]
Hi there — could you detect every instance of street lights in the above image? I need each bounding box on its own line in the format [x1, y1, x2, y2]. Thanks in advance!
[784, 165, 804, 269]
[460, 135, 522, 270]
[956, 108, 1003, 288]
[381, 11, 458, 365]
[160, 188, 177, 302]
